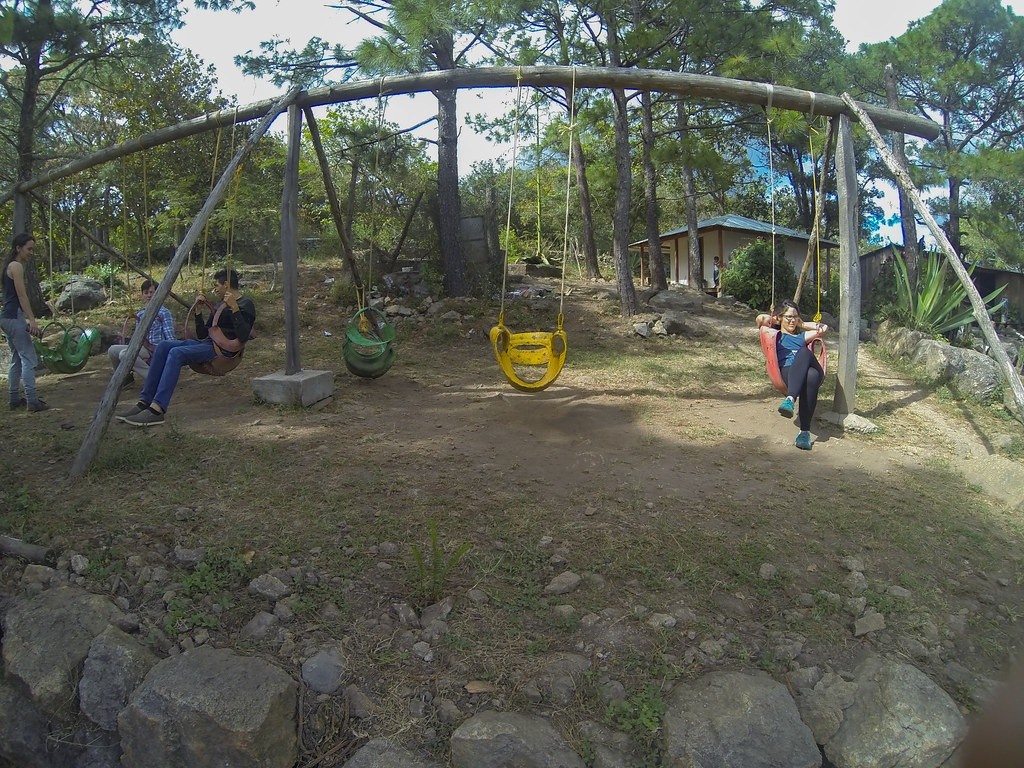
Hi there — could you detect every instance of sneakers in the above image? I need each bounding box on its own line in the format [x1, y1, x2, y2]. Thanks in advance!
[778, 397, 795, 419]
[114, 406, 164, 426]
[795, 431, 812, 450]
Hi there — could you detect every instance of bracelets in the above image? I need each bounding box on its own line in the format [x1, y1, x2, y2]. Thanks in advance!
[231, 305, 239, 310]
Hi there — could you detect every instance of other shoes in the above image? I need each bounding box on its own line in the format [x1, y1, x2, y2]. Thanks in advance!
[122, 377, 134, 390]
[9, 397, 27, 411]
[28, 401, 50, 410]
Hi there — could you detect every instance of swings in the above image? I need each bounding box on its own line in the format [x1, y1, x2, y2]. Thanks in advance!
[119, 150, 167, 355]
[183, 123, 249, 377]
[758, 110, 829, 401]
[33, 175, 102, 375]
[488, 84, 576, 394]
[343, 94, 398, 381]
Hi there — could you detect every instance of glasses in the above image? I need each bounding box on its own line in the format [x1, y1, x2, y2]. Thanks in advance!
[782, 315, 800, 320]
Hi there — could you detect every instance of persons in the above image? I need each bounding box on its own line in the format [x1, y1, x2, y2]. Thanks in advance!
[713, 256, 721, 288]
[0, 233, 49, 411]
[108, 280, 176, 391]
[755, 299, 830, 450]
[114, 269, 256, 426]
